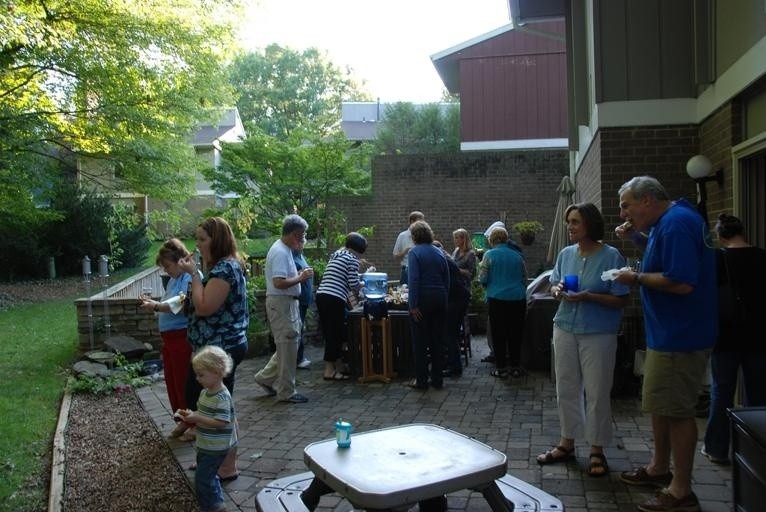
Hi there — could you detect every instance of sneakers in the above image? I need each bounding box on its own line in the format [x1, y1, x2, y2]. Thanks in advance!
[481, 354, 495, 363]
[166, 422, 187, 440]
[281, 394, 308, 403]
[255, 377, 276, 395]
[701, 445, 729, 463]
[297, 361, 312, 368]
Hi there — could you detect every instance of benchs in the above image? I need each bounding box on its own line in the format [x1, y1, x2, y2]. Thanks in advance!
[257, 465, 563, 511]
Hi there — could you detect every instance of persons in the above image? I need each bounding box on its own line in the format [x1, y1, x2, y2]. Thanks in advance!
[431, 239, 471, 377]
[178, 217, 247, 482]
[316, 232, 367, 381]
[713, 212, 766, 464]
[294, 234, 313, 372]
[254, 216, 315, 403]
[614, 174, 718, 512]
[406, 221, 453, 391]
[392, 211, 425, 283]
[451, 227, 479, 286]
[137, 239, 202, 443]
[478, 229, 527, 381]
[482, 220, 523, 255]
[180, 345, 237, 510]
[534, 202, 630, 477]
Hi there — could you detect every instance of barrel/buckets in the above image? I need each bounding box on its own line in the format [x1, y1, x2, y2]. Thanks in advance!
[360, 273, 388, 299]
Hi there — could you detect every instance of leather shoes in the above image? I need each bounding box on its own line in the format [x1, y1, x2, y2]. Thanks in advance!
[619, 466, 673, 488]
[638, 487, 701, 512]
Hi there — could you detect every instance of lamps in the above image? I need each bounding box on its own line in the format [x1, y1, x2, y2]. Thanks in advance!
[686, 155, 726, 203]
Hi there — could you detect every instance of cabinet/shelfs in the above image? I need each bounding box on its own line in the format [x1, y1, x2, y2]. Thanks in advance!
[726, 404, 766, 512]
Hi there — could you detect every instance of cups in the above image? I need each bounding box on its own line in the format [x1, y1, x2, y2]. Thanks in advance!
[564, 274, 579, 293]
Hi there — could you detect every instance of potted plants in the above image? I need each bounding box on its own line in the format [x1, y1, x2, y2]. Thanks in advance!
[513, 218, 543, 248]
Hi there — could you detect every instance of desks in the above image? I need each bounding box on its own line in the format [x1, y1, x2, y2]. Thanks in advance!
[350, 287, 414, 376]
[302, 423, 514, 512]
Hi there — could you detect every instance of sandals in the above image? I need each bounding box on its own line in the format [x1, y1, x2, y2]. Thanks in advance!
[536, 445, 576, 464]
[491, 370, 508, 378]
[587, 452, 608, 477]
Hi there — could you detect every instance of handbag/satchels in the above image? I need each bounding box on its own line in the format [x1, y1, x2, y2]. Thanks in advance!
[718, 246, 747, 329]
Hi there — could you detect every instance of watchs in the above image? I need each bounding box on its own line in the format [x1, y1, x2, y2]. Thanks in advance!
[153, 301, 160, 312]
[634, 273, 641, 284]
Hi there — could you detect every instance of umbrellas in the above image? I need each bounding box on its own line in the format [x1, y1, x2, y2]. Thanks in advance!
[546, 176, 576, 265]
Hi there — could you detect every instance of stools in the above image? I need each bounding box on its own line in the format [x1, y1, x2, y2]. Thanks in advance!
[457, 315, 477, 367]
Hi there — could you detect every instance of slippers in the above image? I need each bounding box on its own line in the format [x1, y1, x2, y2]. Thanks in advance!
[339, 366, 352, 375]
[324, 370, 350, 380]
[216, 473, 238, 483]
[189, 461, 198, 470]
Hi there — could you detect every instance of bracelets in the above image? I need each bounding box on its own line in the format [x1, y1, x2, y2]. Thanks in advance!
[190, 270, 200, 277]
